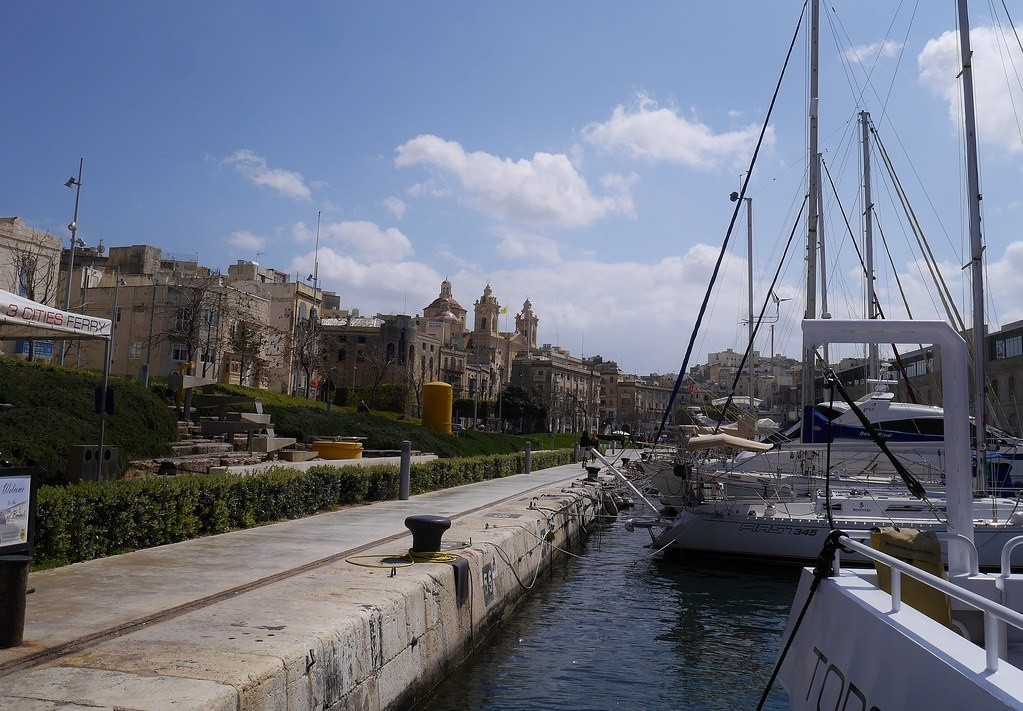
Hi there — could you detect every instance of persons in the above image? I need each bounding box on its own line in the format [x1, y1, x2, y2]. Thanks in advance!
[636, 433, 652, 443]
[580, 430, 590, 462]
[621, 435, 624, 449]
[357, 400, 369, 413]
[590, 433, 598, 462]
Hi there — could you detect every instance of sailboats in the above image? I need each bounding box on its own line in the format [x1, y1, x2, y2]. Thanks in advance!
[582, 0, 1023, 711]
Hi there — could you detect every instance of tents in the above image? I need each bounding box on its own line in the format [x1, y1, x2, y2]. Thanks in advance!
[0, 287, 114, 479]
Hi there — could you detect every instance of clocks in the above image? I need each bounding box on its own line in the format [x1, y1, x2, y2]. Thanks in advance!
[479, 306, 487, 314]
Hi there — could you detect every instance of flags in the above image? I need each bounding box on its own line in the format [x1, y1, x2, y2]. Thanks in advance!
[688, 383, 696, 394]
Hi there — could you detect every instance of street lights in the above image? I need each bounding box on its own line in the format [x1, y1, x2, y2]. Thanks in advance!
[305, 261, 318, 400]
[107, 265, 128, 377]
[57, 157, 86, 367]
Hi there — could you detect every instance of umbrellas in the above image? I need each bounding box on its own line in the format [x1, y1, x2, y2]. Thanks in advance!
[613, 430, 631, 436]
